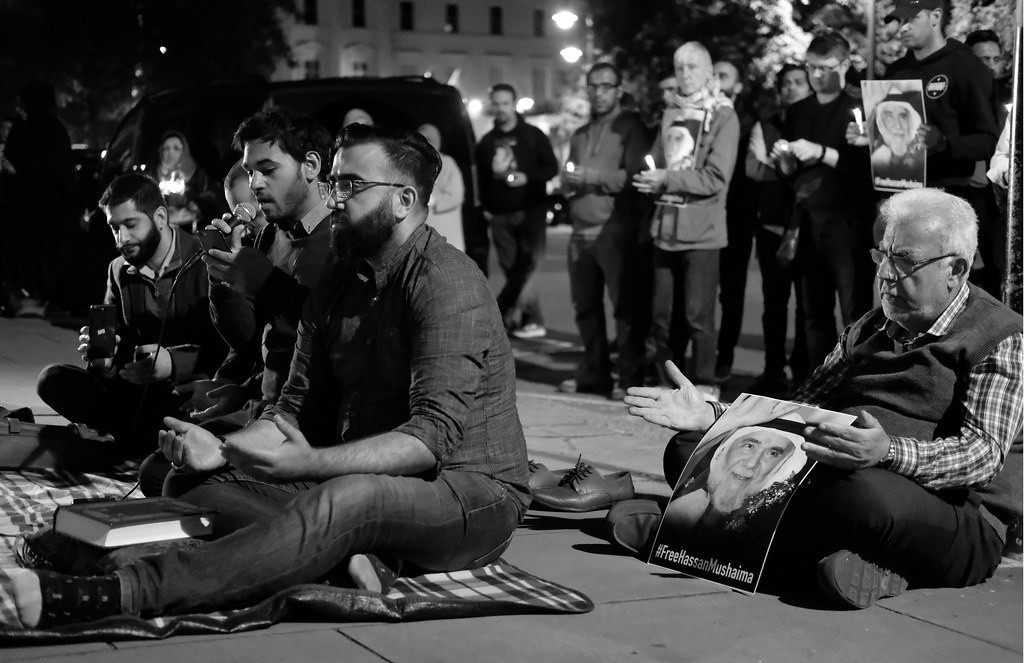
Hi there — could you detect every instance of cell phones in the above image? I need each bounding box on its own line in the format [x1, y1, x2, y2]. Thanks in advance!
[87, 304, 118, 359]
[196, 228, 232, 262]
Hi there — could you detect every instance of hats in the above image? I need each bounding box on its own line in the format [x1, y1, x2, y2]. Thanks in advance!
[884, 0, 944, 24]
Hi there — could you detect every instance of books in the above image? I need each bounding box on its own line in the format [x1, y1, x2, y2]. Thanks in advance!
[52, 494, 220, 550]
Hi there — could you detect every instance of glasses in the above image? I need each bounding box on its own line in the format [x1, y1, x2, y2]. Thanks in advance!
[319, 180, 405, 202]
[869, 249, 958, 274]
[586, 82, 617, 92]
[805, 62, 840, 73]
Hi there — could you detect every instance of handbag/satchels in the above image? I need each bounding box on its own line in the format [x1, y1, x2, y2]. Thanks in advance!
[0, 402, 87, 470]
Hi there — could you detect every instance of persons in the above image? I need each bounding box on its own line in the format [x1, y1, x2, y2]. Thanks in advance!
[869, 87, 925, 179]
[663, 411, 826, 593]
[621, 186, 1024, 610]
[661, 117, 695, 205]
[0, 0, 1013, 635]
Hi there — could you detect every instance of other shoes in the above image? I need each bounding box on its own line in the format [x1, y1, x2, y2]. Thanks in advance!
[513, 323, 546, 338]
[611, 386, 631, 400]
[605, 499, 669, 554]
[560, 378, 588, 393]
[713, 368, 731, 383]
[820, 541, 910, 607]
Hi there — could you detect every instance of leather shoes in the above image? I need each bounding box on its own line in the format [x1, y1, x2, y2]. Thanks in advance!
[528, 461, 576, 491]
[531, 453, 634, 511]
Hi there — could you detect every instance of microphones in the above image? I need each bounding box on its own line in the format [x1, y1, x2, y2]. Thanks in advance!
[175, 202, 256, 281]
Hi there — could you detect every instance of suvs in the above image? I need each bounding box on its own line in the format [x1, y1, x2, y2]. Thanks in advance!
[87, 72, 486, 260]
